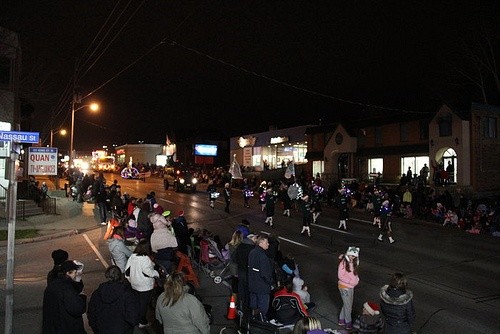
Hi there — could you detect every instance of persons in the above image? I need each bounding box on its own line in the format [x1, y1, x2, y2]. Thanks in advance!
[223, 183, 232, 212]
[264, 188, 276, 226]
[207, 160, 500, 244]
[43, 163, 416, 334]
[337, 246, 360, 330]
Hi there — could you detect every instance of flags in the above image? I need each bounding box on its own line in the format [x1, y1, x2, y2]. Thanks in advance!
[228, 158, 243, 179]
[284, 160, 295, 179]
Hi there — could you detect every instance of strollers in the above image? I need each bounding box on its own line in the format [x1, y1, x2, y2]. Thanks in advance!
[199, 238, 237, 283]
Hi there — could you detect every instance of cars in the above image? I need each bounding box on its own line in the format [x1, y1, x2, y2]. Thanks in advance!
[121, 167, 151, 179]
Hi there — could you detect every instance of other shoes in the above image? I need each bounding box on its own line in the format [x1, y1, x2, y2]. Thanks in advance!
[338, 320, 345, 326]
[378, 234, 383, 241]
[389, 237, 396, 244]
[345, 322, 352, 329]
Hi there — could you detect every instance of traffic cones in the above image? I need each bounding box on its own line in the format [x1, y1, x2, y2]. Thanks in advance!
[226, 294, 237, 320]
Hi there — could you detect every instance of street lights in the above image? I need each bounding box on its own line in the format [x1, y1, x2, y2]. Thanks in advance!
[51, 129, 66, 147]
[68, 104, 99, 176]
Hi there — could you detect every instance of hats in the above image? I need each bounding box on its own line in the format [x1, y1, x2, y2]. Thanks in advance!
[345, 246, 360, 265]
[225, 183, 230, 188]
[363, 301, 379, 316]
[178, 210, 184, 216]
[301, 194, 308, 200]
[154, 203, 165, 215]
[60, 261, 82, 272]
[233, 224, 250, 238]
[162, 210, 170, 216]
[381, 200, 389, 205]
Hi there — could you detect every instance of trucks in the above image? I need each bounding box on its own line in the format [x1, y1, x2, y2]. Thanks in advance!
[92, 156, 115, 171]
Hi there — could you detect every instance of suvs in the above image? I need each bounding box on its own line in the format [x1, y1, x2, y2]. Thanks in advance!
[164, 166, 197, 192]
